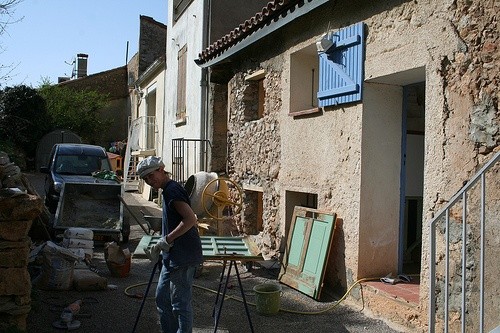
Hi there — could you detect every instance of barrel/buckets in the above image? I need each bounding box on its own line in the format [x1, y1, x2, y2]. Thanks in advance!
[253, 284, 282, 316]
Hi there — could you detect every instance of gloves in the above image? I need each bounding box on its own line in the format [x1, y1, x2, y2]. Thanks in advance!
[148, 235, 174, 261]
[380, 273, 411, 285]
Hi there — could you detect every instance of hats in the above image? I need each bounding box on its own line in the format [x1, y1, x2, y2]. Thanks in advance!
[137, 157, 165, 178]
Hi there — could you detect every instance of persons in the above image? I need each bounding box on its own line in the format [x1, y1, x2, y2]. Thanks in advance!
[136, 157, 204, 333]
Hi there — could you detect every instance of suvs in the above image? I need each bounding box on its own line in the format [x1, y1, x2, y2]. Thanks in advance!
[40, 143, 124, 216]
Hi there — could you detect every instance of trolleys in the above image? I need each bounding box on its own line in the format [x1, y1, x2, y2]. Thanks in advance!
[139, 210, 162, 236]
[48, 181, 150, 243]
